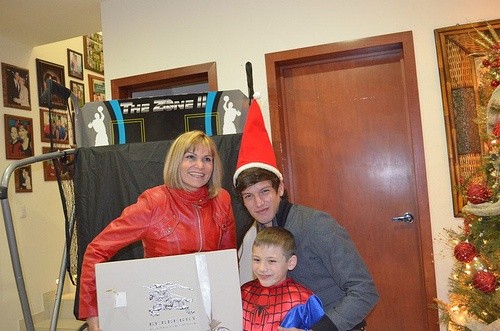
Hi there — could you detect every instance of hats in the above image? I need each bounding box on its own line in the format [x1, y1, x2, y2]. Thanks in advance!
[232, 91, 283, 188]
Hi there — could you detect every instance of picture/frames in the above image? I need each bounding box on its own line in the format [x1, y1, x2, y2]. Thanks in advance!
[87, 74, 106, 103]
[67, 48, 84, 80]
[35, 57, 68, 111]
[433, 20, 500, 217]
[14, 164, 33, 193]
[42, 146, 74, 181]
[69, 79, 85, 111]
[83, 33, 104, 75]
[39, 108, 69, 144]
[1, 62, 32, 111]
[4, 114, 34, 160]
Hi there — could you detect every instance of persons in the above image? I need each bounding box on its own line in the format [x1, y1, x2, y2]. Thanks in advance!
[44, 118, 67, 143]
[78, 131, 237, 331]
[234, 95, 379, 331]
[7, 122, 33, 157]
[9, 71, 30, 107]
[240, 226, 325, 331]
[18, 168, 31, 190]
[48, 156, 74, 179]
[44, 73, 62, 107]
